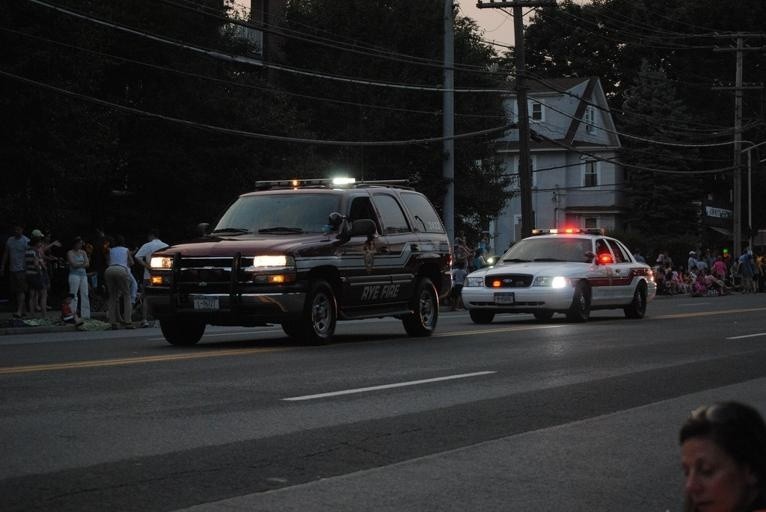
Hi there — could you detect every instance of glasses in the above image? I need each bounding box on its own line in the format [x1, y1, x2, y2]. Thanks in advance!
[687, 404, 736, 424]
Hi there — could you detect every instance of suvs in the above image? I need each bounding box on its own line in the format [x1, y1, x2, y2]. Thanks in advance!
[138, 170, 461, 350]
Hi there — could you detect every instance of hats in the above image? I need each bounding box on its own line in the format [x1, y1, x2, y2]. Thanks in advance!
[31, 229, 44, 237]
[689, 251, 696, 255]
[473, 248, 486, 259]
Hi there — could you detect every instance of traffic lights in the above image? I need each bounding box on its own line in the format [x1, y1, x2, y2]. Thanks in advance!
[723, 245, 730, 261]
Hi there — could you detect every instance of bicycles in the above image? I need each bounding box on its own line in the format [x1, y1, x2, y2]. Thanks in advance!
[115, 283, 143, 323]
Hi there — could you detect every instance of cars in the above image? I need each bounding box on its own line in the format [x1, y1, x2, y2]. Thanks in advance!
[459, 222, 660, 327]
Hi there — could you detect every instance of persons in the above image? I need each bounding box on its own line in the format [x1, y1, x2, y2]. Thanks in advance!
[674, 399, 766, 512]
[0, 219, 171, 333]
[452, 228, 517, 314]
[634, 247, 766, 298]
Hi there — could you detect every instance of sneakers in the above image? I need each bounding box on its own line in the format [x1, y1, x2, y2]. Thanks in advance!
[112, 320, 149, 330]
[75, 321, 84, 327]
